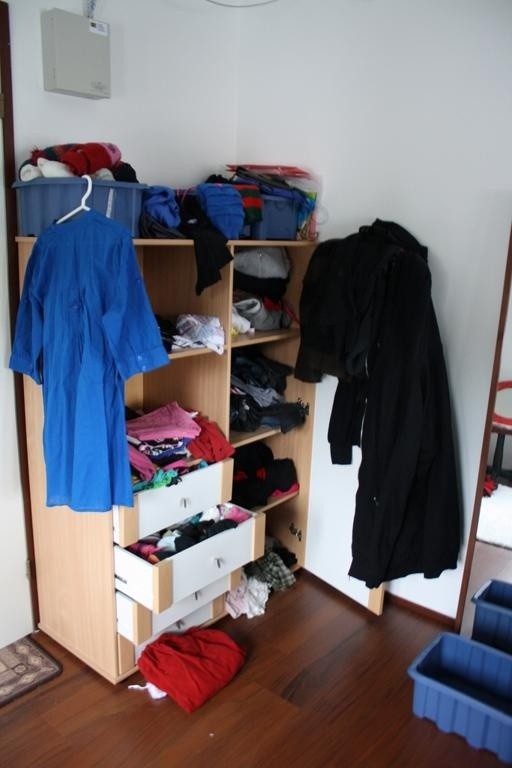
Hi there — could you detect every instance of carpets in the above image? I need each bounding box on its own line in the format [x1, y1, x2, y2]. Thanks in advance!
[476, 484, 512, 550]
[0, 636, 63, 710]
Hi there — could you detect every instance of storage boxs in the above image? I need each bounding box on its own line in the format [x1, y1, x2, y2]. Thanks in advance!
[472, 578, 512, 655]
[173, 190, 300, 240]
[11, 180, 148, 235]
[409, 631, 512, 765]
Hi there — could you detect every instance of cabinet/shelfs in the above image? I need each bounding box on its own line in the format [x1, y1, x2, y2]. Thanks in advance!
[13, 236, 386, 685]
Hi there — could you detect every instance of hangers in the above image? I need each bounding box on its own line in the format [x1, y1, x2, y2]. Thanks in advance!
[52, 174, 93, 227]
